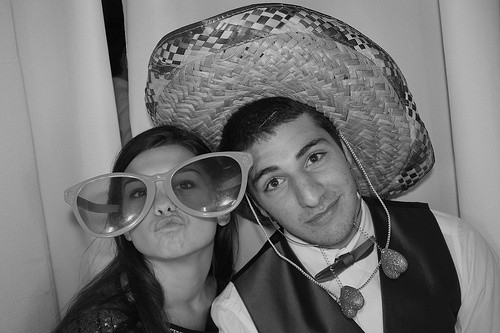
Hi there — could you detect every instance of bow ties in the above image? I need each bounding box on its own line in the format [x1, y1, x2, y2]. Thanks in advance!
[311, 237, 373, 289]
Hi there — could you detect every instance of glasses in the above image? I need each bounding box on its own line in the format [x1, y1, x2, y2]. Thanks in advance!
[61, 148, 254, 238]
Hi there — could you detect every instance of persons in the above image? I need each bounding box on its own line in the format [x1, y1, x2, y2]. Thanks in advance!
[47, 121, 241, 333]
[216, 94, 500, 333]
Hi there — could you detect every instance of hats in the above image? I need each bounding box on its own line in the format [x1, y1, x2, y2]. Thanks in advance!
[143, 1, 435, 202]
